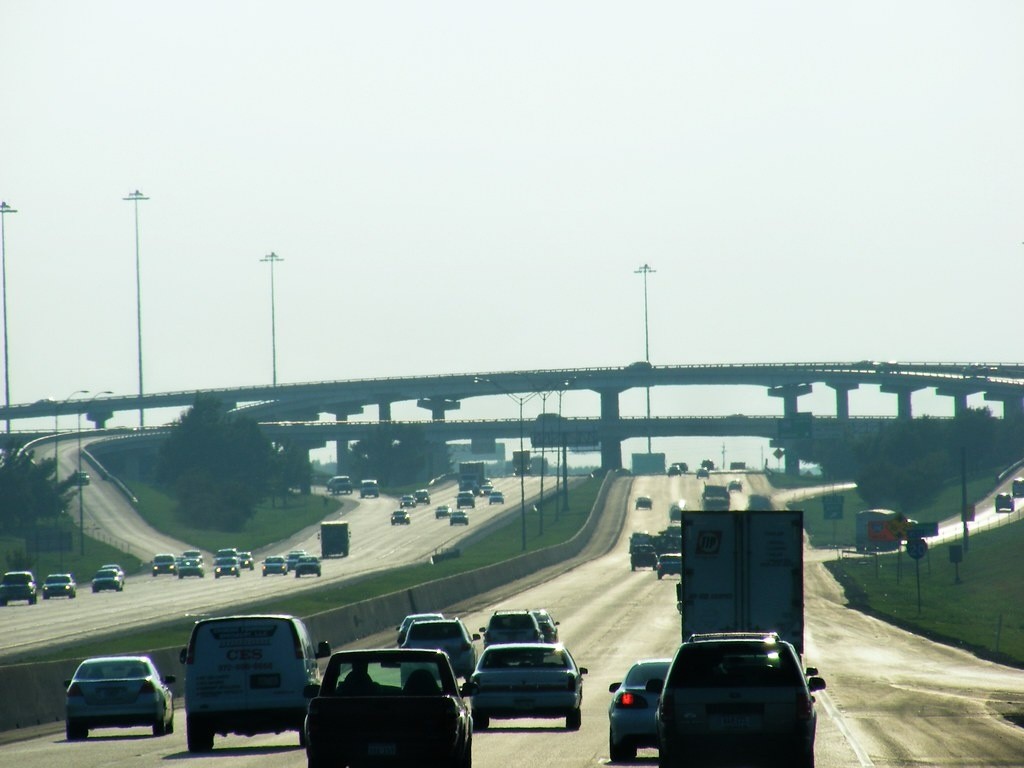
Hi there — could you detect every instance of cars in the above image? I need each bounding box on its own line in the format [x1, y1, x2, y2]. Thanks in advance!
[466, 642, 588, 730]
[605, 658, 674, 759]
[62, 655, 178, 741]
[41, 573, 77, 600]
[91, 569, 123, 594]
[77, 470, 90, 485]
[325, 461, 506, 527]
[626, 460, 747, 581]
[528, 608, 561, 655]
[395, 612, 446, 648]
[149, 547, 322, 578]
[101, 564, 126, 585]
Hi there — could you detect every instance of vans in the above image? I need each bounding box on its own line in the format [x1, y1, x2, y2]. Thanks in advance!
[178, 611, 332, 754]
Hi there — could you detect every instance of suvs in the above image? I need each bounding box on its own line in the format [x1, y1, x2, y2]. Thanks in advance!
[994, 492, 1015, 512]
[398, 616, 482, 687]
[478, 608, 546, 663]
[1011, 476, 1024, 497]
[643, 628, 826, 768]
[0, 571, 38, 607]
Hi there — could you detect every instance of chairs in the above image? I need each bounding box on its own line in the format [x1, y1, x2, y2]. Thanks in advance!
[339, 670, 386, 694]
[404, 670, 443, 698]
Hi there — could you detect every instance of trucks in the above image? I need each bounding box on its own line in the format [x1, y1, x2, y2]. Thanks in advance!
[855, 508, 899, 552]
[674, 509, 808, 663]
[511, 450, 534, 476]
[316, 520, 351, 558]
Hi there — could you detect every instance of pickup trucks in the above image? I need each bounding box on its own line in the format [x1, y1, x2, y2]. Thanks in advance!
[301, 649, 478, 768]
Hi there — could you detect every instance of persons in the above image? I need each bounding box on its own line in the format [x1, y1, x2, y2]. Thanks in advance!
[91, 667, 104, 678]
[340, 664, 381, 690]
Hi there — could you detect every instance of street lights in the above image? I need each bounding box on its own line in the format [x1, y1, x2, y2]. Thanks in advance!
[120, 189, 152, 433]
[0, 200, 18, 458]
[53, 388, 90, 526]
[473, 376, 570, 552]
[631, 262, 659, 471]
[259, 252, 285, 402]
[513, 371, 578, 534]
[78, 390, 115, 555]
